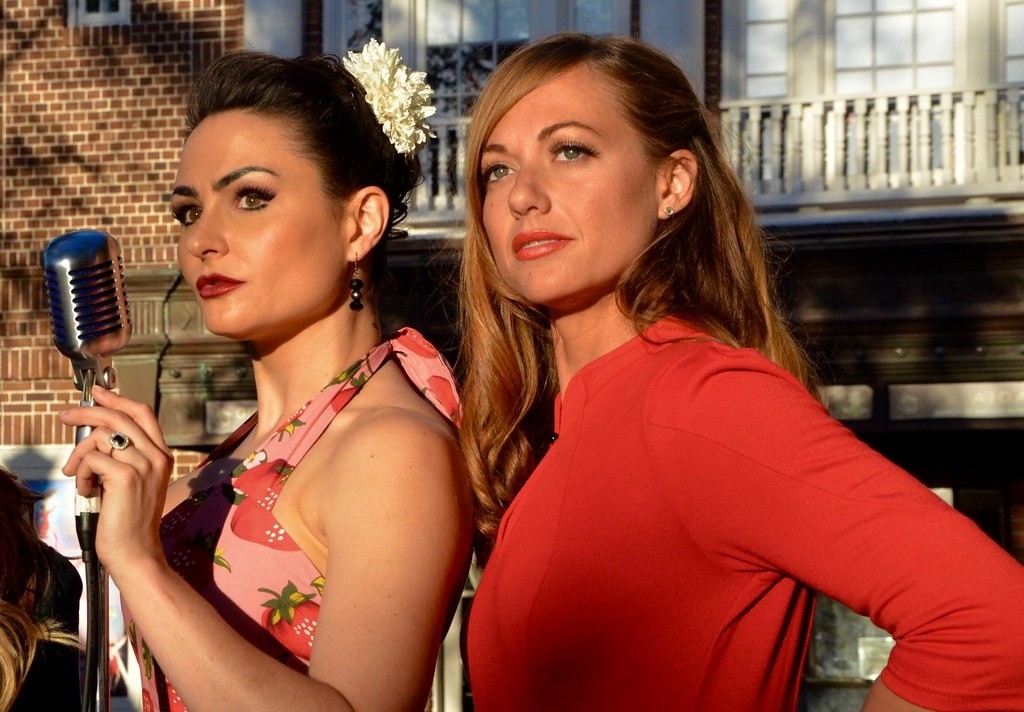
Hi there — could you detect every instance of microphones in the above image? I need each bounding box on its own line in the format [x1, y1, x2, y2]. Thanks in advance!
[43, 228, 132, 561]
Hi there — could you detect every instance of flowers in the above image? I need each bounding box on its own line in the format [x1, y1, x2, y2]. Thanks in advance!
[342, 35, 439, 162]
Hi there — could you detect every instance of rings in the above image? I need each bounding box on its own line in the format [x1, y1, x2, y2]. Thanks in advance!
[104, 432, 129, 456]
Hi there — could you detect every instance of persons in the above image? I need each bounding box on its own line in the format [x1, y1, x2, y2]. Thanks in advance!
[436, 33, 1024, 712]
[62, 39, 476, 712]
[0, 465, 83, 712]
